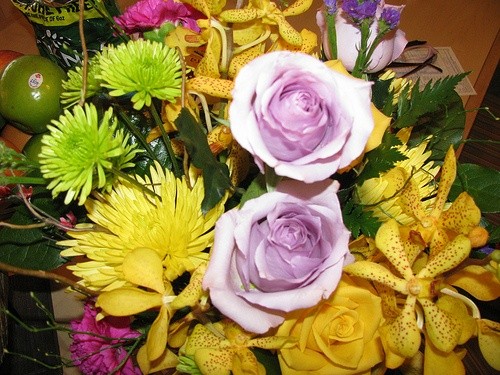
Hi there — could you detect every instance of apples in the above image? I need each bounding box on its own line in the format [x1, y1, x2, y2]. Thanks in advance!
[0, 48, 24, 74]
[21, 131, 50, 163]
[0, 55, 70, 135]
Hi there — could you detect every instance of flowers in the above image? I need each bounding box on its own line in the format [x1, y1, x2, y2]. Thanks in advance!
[0, 0, 500, 375]
